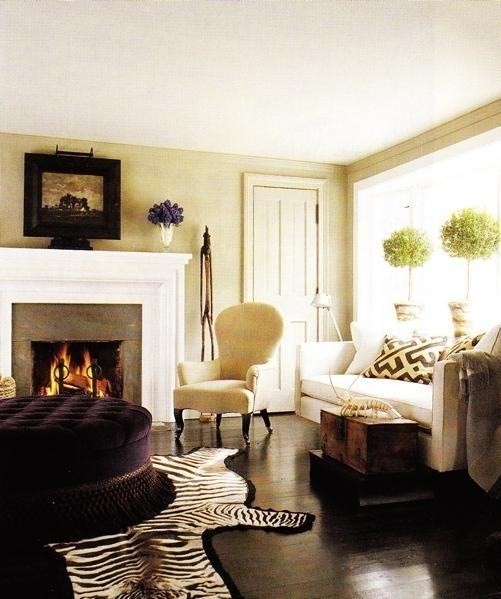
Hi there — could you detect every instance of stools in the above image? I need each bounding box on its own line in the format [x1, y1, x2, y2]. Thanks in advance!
[0, 393, 175, 543]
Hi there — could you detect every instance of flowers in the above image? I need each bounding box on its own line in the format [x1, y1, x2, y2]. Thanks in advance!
[148, 199, 184, 229]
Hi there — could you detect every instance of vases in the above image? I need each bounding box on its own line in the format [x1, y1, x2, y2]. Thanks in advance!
[159, 222, 175, 253]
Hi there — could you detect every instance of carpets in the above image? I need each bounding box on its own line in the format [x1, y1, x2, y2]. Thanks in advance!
[1, 448, 314, 599]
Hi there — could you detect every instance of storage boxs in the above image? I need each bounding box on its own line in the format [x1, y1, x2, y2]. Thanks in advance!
[320, 405, 420, 476]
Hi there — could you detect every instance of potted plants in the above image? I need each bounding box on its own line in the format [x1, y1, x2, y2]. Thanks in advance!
[383, 226, 431, 321]
[440, 207, 501, 326]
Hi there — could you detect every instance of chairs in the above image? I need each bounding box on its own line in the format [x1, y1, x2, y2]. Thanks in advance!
[174, 302, 284, 448]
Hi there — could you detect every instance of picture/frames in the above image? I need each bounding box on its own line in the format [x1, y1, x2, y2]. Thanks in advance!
[24, 152, 121, 241]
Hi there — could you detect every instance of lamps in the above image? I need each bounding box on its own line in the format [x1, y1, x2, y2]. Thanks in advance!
[313, 293, 345, 342]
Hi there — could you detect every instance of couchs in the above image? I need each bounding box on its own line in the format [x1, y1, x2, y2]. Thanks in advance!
[301, 319, 486, 474]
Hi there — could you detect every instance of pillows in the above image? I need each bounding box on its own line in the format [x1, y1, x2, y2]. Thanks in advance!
[343, 321, 501, 386]
